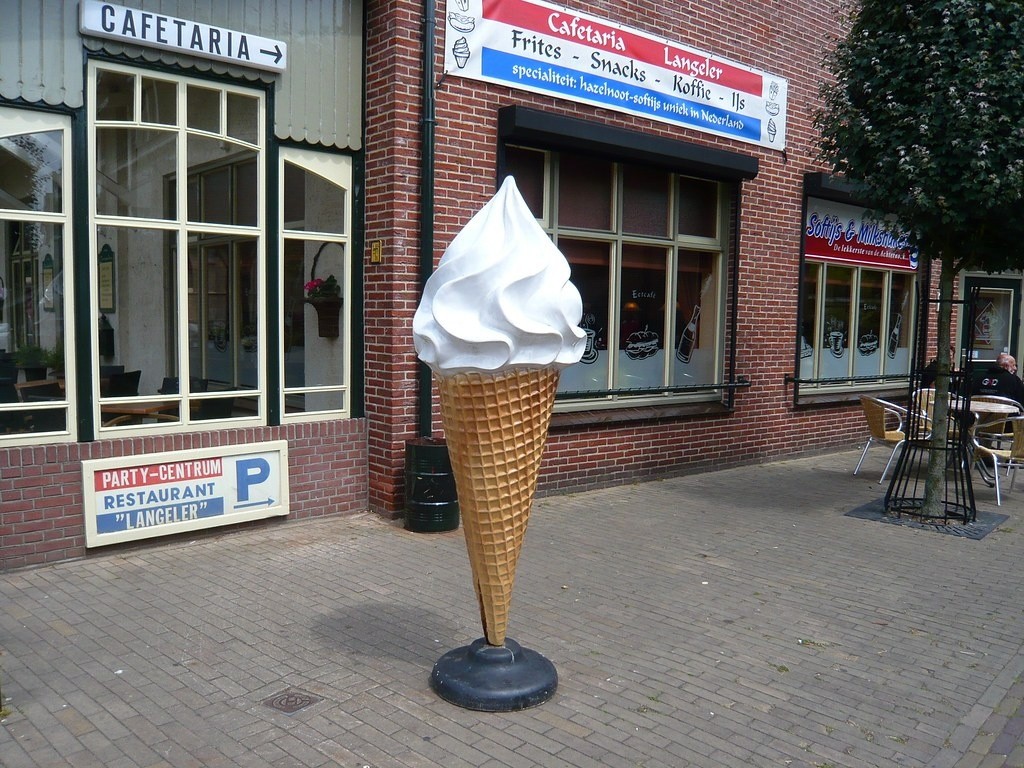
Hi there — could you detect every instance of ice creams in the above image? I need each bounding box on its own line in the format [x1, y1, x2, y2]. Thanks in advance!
[413, 175, 587, 646]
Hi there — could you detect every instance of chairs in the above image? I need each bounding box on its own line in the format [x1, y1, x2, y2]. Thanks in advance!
[853, 395, 935, 483]
[965, 415, 1024, 505]
[912, 387, 1024, 487]
[0, 348, 238, 435]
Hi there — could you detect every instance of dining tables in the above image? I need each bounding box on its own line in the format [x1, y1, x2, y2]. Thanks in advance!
[929, 400, 1018, 488]
[100, 399, 200, 428]
[14, 377, 110, 398]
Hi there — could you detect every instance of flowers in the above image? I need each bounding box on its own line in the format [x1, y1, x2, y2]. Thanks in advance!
[304, 275, 340, 300]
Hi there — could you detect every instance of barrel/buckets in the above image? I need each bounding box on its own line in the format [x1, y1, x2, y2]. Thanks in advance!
[403, 435, 461, 535]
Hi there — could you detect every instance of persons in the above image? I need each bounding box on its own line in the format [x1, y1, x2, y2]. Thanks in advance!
[970, 354, 1024, 433]
[920, 345, 956, 394]
